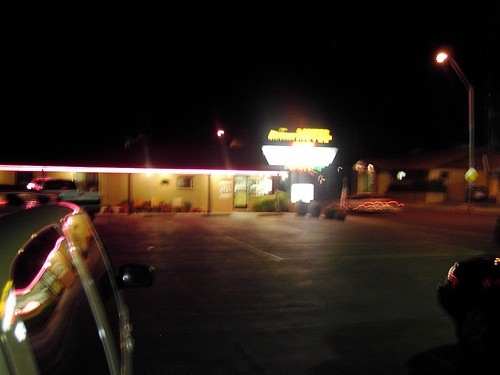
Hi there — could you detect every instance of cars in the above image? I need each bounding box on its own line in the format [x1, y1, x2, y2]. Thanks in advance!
[0, 190, 157, 375]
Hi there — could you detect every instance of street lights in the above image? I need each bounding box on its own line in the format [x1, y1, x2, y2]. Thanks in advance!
[435, 51, 476, 206]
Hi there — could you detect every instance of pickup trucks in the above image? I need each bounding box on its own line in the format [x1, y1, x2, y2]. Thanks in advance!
[26, 179, 102, 222]
